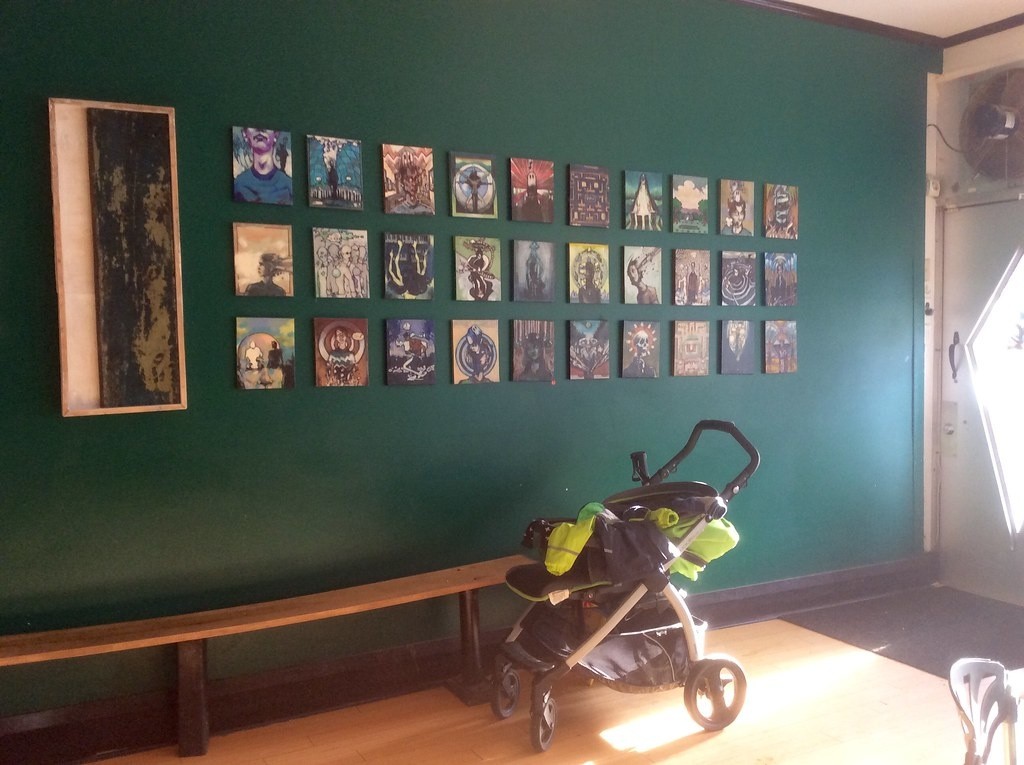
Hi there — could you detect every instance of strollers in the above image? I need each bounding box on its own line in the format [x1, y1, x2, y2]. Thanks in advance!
[492, 420, 760, 752]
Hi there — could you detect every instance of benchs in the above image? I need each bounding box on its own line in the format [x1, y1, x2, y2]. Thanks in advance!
[1, 556, 540, 756]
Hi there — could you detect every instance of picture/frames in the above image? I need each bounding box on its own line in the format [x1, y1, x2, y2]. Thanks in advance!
[449, 149, 499, 219]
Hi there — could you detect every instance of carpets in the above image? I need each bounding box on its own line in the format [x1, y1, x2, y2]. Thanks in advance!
[781, 586, 1024, 683]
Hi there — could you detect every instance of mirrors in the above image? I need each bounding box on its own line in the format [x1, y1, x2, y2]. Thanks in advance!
[50, 98, 187, 417]
